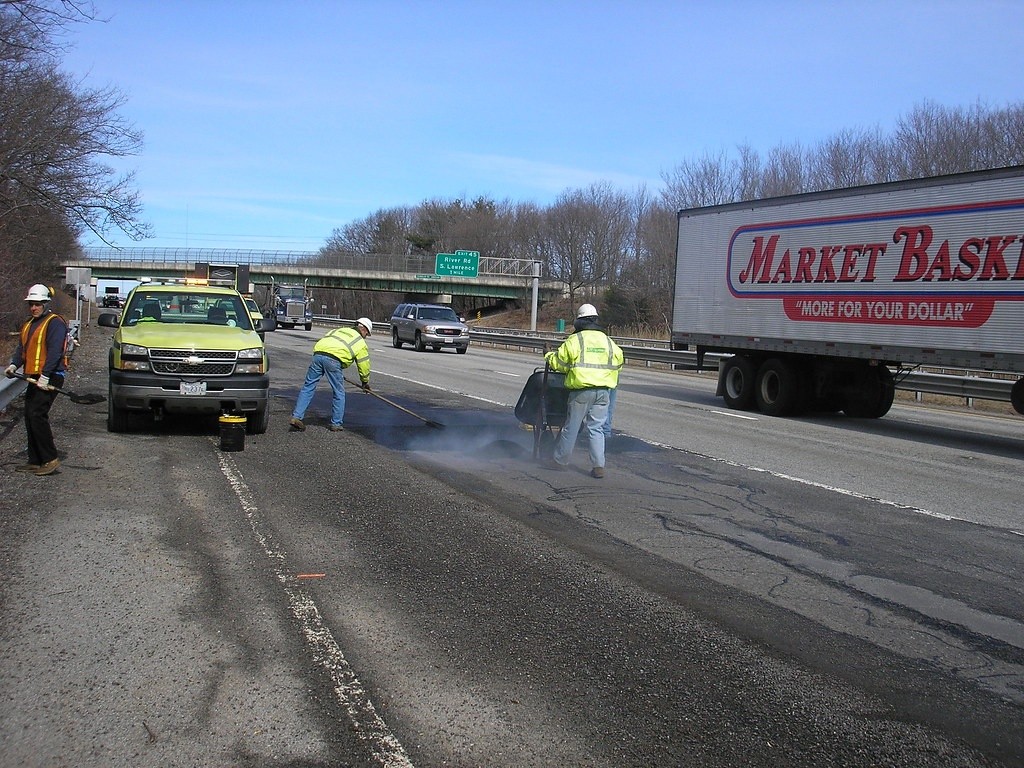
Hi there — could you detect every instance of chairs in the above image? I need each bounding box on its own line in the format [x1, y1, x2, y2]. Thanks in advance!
[207, 307, 226, 321]
[143, 303, 161, 318]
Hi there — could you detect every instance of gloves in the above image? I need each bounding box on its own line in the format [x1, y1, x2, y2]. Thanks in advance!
[4, 364, 16, 379]
[543, 342, 552, 355]
[362, 381, 372, 394]
[37, 374, 49, 390]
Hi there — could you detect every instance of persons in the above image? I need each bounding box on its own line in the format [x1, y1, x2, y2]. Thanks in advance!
[544, 303, 624, 478]
[5, 284, 68, 475]
[290, 318, 373, 432]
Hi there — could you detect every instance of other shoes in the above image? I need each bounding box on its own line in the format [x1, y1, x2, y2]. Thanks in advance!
[542, 460, 568, 472]
[290, 417, 306, 429]
[591, 467, 604, 478]
[331, 424, 345, 431]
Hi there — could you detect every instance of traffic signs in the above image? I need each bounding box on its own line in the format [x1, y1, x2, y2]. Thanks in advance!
[435, 249, 480, 278]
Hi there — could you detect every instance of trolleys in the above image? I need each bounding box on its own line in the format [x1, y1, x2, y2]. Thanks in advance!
[514, 359, 585, 461]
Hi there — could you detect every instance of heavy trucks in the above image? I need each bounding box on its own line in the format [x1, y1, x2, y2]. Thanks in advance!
[265, 276, 315, 331]
[669, 163, 1024, 419]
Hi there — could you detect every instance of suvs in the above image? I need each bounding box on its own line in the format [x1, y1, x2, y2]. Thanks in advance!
[390, 301, 470, 354]
[215, 297, 266, 340]
[97, 280, 270, 435]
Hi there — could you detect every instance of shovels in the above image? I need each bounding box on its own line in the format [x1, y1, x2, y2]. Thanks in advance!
[343, 377, 447, 430]
[6, 371, 107, 405]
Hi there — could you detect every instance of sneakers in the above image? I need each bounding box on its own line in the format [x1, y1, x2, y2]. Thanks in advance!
[15, 463, 43, 472]
[34, 457, 60, 475]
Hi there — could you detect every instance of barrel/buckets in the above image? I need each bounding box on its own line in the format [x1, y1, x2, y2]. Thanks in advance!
[218, 416, 247, 452]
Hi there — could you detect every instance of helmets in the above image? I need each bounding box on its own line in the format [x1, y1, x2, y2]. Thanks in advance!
[576, 303, 599, 319]
[23, 283, 51, 301]
[356, 318, 373, 337]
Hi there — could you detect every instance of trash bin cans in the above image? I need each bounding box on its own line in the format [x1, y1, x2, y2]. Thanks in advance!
[556, 319, 564, 332]
